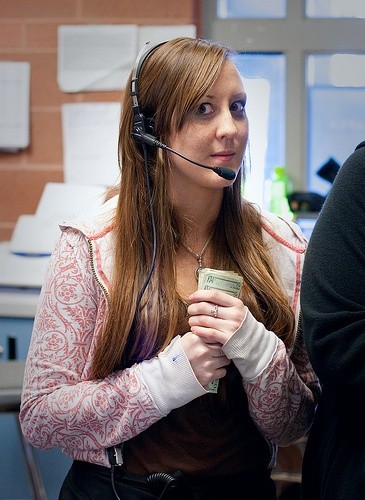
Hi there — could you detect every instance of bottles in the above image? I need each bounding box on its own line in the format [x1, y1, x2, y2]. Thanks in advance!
[265, 168, 292, 220]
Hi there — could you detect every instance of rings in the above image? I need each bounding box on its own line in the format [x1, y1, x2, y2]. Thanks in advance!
[211, 305, 218, 317]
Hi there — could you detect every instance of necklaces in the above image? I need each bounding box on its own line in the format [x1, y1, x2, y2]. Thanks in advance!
[179, 228, 216, 279]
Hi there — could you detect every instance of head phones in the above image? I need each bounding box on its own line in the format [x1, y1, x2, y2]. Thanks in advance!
[132, 41, 170, 160]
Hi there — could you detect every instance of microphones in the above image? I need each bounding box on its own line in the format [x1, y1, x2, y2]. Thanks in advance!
[142, 134, 236, 181]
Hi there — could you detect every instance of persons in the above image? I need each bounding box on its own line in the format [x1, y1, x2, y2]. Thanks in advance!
[19, 38, 321, 500]
[301, 141, 365, 500]
